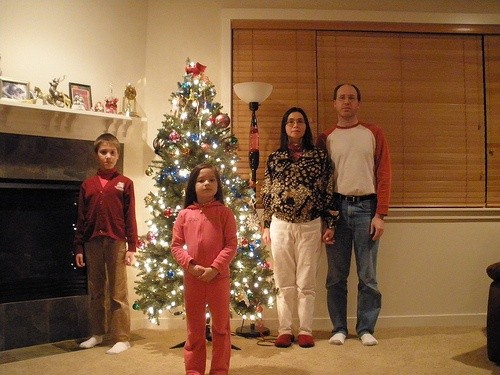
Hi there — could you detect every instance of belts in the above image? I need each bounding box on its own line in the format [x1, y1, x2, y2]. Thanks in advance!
[333, 192, 377, 203]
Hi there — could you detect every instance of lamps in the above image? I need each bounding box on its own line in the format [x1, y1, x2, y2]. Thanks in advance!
[234, 81, 271, 340]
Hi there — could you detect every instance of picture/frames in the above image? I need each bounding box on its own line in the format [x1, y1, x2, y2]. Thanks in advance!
[70, 82, 92, 110]
[0, 75, 31, 103]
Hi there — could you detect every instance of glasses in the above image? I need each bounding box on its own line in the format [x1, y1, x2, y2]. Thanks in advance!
[285, 120, 305, 126]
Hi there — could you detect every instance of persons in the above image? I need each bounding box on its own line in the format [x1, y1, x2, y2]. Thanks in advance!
[75, 133, 138, 354]
[171, 164, 237, 375]
[263, 107, 339, 348]
[316, 83, 391, 346]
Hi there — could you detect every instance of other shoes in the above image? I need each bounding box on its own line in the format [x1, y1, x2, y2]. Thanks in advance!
[298, 334, 314, 347]
[275, 333, 295, 347]
